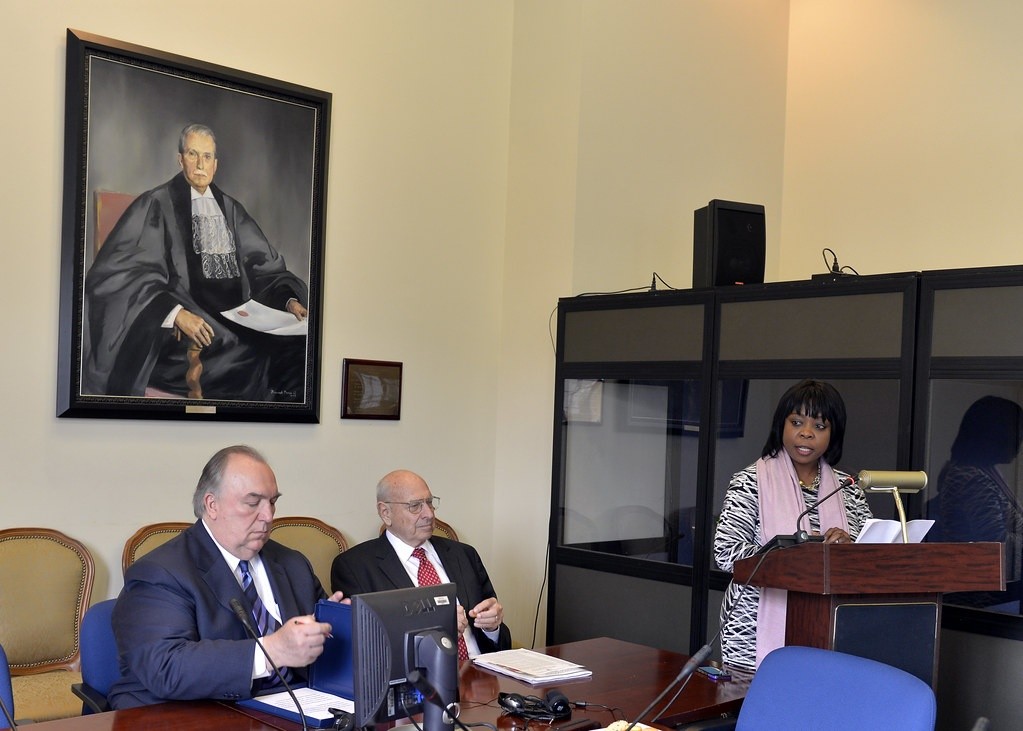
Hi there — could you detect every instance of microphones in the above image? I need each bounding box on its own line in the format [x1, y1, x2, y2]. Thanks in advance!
[229, 597, 308, 731]
[755, 473, 860, 555]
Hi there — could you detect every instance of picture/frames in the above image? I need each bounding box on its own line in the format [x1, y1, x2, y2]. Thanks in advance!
[55, 29, 332, 427]
[340, 357, 406, 420]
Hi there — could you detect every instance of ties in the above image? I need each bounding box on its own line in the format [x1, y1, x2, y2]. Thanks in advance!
[411, 548, 469, 669]
[238, 560, 293, 686]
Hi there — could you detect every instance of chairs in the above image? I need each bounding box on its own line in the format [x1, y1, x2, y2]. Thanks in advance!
[121, 522, 193, 577]
[270, 515, 347, 597]
[379, 516, 459, 544]
[70, 597, 118, 714]
[735, 645, 937, 731]
[0, 528, 95, 724]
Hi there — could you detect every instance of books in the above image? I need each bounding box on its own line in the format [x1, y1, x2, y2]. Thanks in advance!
[474, 648, 593, 683]
[855, 519, 935, 543]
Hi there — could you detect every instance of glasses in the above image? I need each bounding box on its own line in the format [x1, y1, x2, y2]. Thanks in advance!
[383, 496, 440, 514]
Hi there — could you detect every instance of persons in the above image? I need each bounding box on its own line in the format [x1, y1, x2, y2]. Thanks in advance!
[714, 380, 874, 678]
[107, 445, 351, 709]
[937, 398, 1023, 614]
[330, 471, 512, 661]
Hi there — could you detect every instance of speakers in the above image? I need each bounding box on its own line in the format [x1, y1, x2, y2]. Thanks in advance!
[692, 199, 766, 288]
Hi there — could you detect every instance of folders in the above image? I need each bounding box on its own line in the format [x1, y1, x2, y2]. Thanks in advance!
[236, 600, 353, 728]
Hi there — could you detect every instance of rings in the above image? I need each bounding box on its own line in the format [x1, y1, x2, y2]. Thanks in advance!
[496, 616, 498, 622]
[835, 540, 838, 543]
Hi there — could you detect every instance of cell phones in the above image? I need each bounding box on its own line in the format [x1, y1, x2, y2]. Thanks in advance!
[698, 667, 731, 680]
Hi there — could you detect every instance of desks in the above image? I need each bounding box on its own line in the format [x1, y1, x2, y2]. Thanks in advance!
[218, 637, 750, 731]
[0, 696, 280, 731]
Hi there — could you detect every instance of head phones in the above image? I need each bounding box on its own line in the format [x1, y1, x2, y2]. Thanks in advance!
[498, 689, 572, 723]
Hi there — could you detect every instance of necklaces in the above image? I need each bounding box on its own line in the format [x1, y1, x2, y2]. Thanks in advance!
[800, 462, 821, 491]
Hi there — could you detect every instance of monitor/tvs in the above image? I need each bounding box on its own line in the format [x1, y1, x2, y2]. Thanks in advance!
[351, 582, 461, 731]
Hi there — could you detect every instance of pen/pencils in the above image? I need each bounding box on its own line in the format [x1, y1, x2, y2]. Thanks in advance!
[294, 620, 334, 637]
